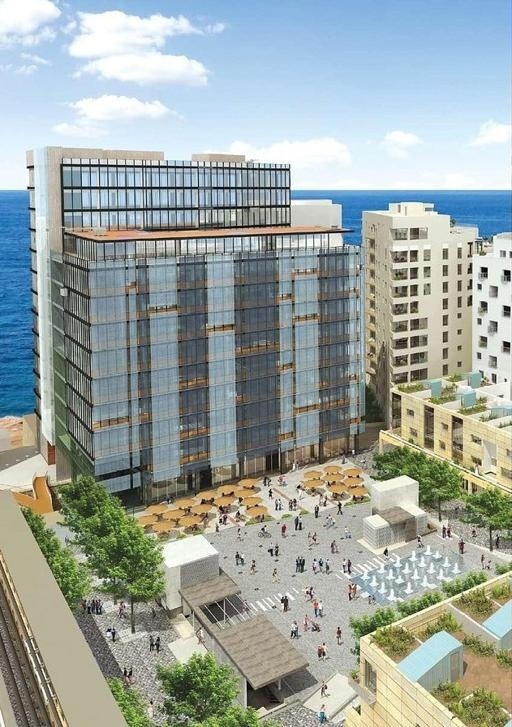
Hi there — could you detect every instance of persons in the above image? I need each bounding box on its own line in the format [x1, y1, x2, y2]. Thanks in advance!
[83, 599, 102, 615]
[151, 607, 156, 619]
[81, 451, 502, 724]
